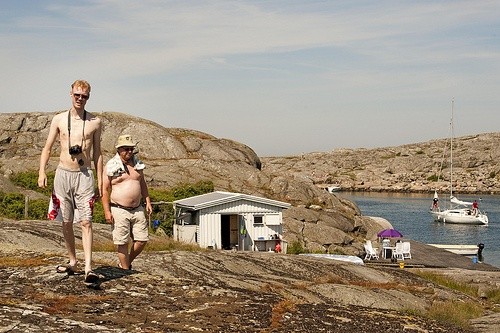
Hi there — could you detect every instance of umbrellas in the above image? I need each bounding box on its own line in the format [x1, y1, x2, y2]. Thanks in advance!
[377, 228, 403, 246]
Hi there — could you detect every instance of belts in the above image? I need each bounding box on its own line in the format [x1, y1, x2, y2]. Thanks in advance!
[109, 202, 141, 211]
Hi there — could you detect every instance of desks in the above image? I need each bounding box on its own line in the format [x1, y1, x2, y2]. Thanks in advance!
[382, 246, 396, 260]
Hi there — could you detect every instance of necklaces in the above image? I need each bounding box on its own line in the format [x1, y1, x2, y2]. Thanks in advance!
[70, 111, 84, 120]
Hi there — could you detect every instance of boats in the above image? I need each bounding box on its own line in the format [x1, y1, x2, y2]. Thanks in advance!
[426, 242, 479, 256]
[325, 186, 341, 191]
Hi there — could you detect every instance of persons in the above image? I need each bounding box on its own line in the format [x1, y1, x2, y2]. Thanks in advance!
[473, 200, 478, 218]
[37, 79, 104, 283]
[103, 135, 153, 271]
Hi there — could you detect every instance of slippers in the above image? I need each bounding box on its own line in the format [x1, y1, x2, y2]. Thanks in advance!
[84, 271, 98, 283]
[54, 263, 75, 273]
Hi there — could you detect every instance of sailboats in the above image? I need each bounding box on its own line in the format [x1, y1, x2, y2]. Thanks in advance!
[428, 100, 490, 225]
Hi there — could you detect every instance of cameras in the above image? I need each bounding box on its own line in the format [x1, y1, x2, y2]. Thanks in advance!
[69, 145, 82, 154]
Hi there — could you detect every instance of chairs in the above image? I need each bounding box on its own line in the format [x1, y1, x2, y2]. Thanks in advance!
[363, 239, 412, 262]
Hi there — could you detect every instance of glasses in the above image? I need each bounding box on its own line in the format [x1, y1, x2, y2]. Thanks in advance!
[121, 147, 135, 151]
[72, 92, 89, 100]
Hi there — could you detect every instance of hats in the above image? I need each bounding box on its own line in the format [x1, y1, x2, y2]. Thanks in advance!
[115, 134, 136, 149]
